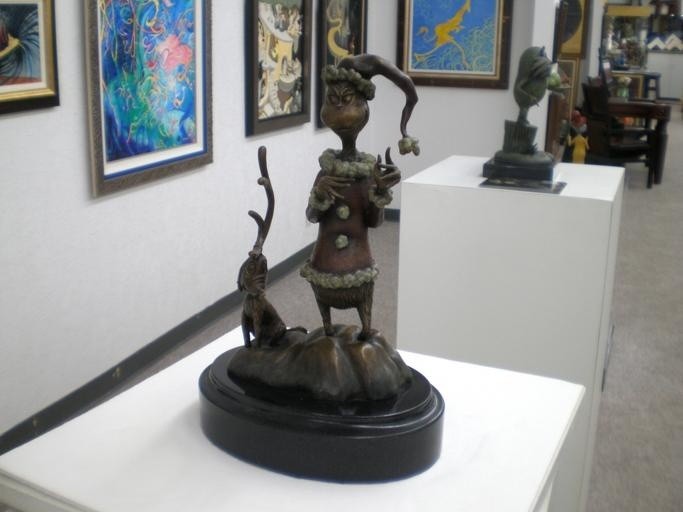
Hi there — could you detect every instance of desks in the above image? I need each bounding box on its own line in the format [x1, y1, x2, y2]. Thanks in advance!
[575, 96, 670, 184]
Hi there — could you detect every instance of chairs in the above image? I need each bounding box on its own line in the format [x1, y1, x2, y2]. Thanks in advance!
[581, 73, 655, 188]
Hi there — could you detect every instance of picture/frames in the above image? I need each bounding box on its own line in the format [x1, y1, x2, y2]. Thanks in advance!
[245, 0, 313, 138]
[0, 0, 61, 115]
[316, 0, 368, 129]
[396, 0, 513, 90]
[83, 0, 213, 199]
